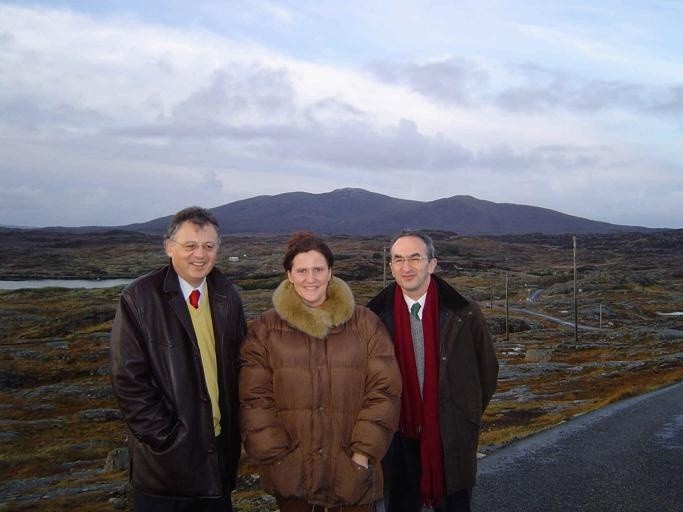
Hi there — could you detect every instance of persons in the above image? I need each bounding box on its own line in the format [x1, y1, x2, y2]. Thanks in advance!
[239, 233, 403, 512]
[365, 229, 498, 512]
[108, 205, 247, 509]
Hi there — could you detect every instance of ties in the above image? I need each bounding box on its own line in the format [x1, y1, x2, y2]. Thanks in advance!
[410, 303, 422, 321]
[189, 289, 201, 310]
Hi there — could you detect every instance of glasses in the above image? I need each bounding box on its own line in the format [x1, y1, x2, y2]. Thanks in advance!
[171, 240, 220, 251]
[390, 255, 439, 264]
